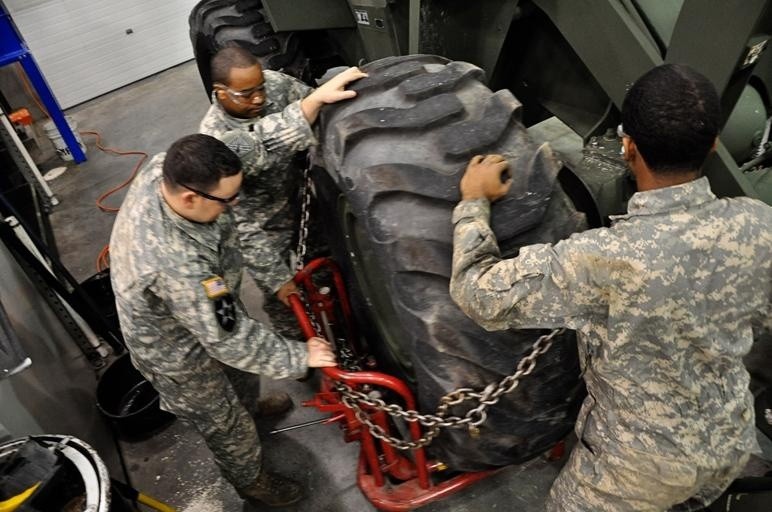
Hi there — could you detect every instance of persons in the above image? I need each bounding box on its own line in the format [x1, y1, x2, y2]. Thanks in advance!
[107, 65, 370, 510]
[450, 60, 771, 510]
[190, 43, 326, 385]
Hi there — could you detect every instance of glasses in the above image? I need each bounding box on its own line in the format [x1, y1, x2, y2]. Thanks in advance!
[175, 178, 239, 205]
[219, 83, 265, 104]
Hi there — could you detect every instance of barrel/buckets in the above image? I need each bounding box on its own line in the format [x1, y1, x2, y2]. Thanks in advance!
[0, 434, 111, 512]
[43, 116, 87, 161]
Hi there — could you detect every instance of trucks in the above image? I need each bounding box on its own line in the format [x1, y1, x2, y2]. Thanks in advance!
[190, 1, 771, 473]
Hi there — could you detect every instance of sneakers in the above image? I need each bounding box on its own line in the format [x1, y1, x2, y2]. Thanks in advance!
[249, 392, 290, 420]
[234, 471, 305, 507]
